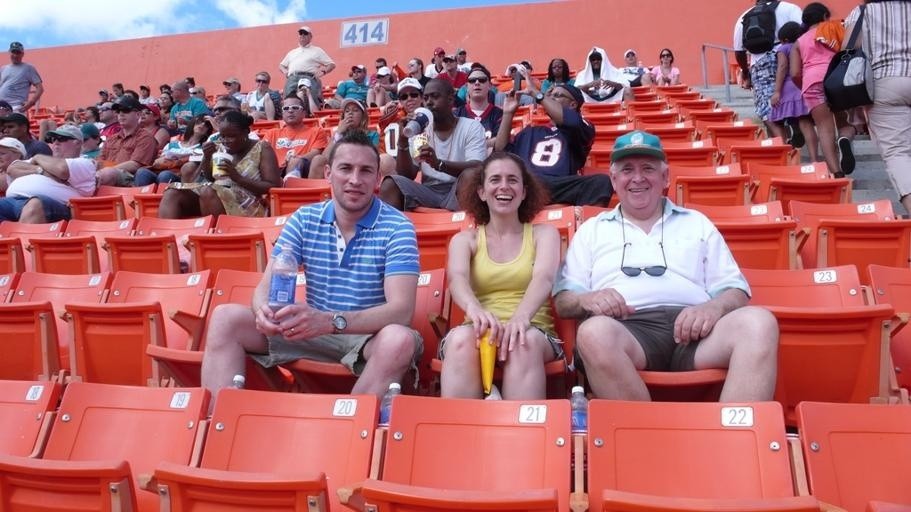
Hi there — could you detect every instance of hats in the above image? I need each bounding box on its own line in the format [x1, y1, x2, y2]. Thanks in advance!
[555, 81, 585, 106]
[298, 26, 313, 34]
[46, 123, 84, 141]
[1, 113, 30, 126]
[80, 122, 99, 138]
[111, 97, 142, 111]
[98, 78, 313, 94]
[519, 47, 639, 73]
[9, 42, 24, 52]
[0, 136, 26, 157]
[143, 102, 161, 117]
[396, 77, 422, 93]
[350, 47, 468, 76]
[612, 130, 666, 161]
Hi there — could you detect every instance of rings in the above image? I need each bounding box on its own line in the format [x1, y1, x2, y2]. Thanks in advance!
[287, 327, 295, 335]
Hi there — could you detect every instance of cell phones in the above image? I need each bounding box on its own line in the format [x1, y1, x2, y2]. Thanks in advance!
[512, 70, 521, 93]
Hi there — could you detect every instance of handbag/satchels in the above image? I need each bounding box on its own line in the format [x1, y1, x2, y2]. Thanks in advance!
[824, 47, 875, 111]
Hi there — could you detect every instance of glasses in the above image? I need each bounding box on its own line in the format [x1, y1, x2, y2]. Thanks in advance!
[115, 107, 133, 113]
[621, 242, 670, 276]
[398, 92, 419, 99]
[282, 105, 303, 111]
[468, 76, 488, 83]
[47, 136, 68, 142]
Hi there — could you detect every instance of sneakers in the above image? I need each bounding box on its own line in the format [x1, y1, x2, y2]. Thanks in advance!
[788, 117, 804, 148]
[838, 135, 856, 174]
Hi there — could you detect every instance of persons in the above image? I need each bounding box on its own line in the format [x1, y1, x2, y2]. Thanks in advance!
[841, 0, 911, 219]
[770, 21, 818, 162]
[0, 25, 681, 273]
[789, 2, 856, 179]
[732, 0, 804, 139]
[552, 130, 780, 401]
[199, 131, 426, 399]
[436, 150, 565, 400]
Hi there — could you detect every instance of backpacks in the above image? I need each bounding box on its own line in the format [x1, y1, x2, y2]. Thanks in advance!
[741, 0, 777, 53]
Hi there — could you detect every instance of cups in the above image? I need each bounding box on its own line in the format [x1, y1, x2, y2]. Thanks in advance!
[402, 107, 433, 137]
[408, 135, 427, 160]
[212, 153, 234, 177]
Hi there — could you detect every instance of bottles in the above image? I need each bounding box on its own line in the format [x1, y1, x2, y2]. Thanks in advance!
[266, 242, 298, 326]
[377, 382, 403, 425]
[568, 386, 591, 435]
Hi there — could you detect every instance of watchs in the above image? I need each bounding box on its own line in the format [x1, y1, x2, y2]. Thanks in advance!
[332, 311, 347, 335]
